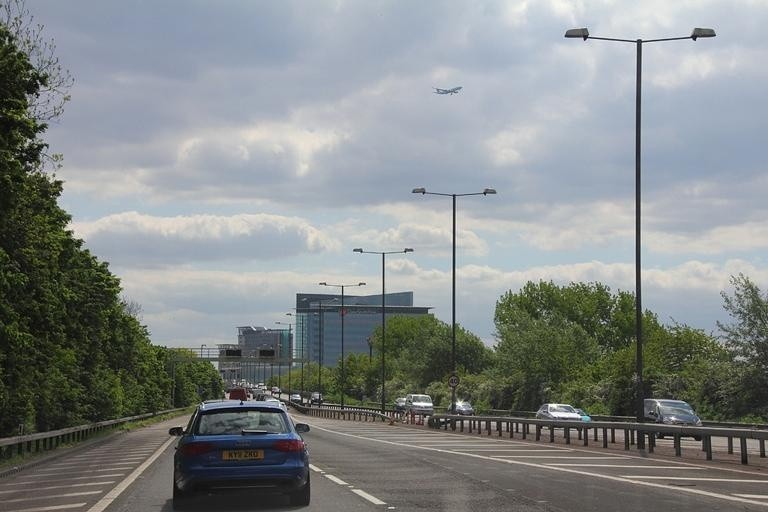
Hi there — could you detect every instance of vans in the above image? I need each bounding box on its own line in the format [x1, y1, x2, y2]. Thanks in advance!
[406, 393, 433, 416]
[643, 399, 704, 441]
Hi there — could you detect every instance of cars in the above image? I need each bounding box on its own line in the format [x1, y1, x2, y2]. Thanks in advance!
[225, 379, 252, 410]
[447, 401, 475, 417]
[393, 397, 407, 413]
[536, 403, 582, 429]
[575, 408, 592, 422]
[168, 399, 313, 510]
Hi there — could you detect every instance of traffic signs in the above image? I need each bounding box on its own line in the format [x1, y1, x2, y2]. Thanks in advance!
[448, 375, 459, 387]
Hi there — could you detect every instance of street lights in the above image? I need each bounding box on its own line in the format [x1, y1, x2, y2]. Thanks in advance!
[201, 344, 206, 357]
[410, 185, 498, 427]
[301, 294, 339, 408]
[247, 309, 318, 406]
[566, 24, 715, 452]
[319, 282, 366, 408]
[353, 248, 415, 421]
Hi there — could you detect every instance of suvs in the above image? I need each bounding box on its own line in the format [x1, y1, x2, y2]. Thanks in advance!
[311, 390, 324, 405]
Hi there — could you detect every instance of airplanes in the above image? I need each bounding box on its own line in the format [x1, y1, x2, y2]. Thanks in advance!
[432, 86, 463, 96]
